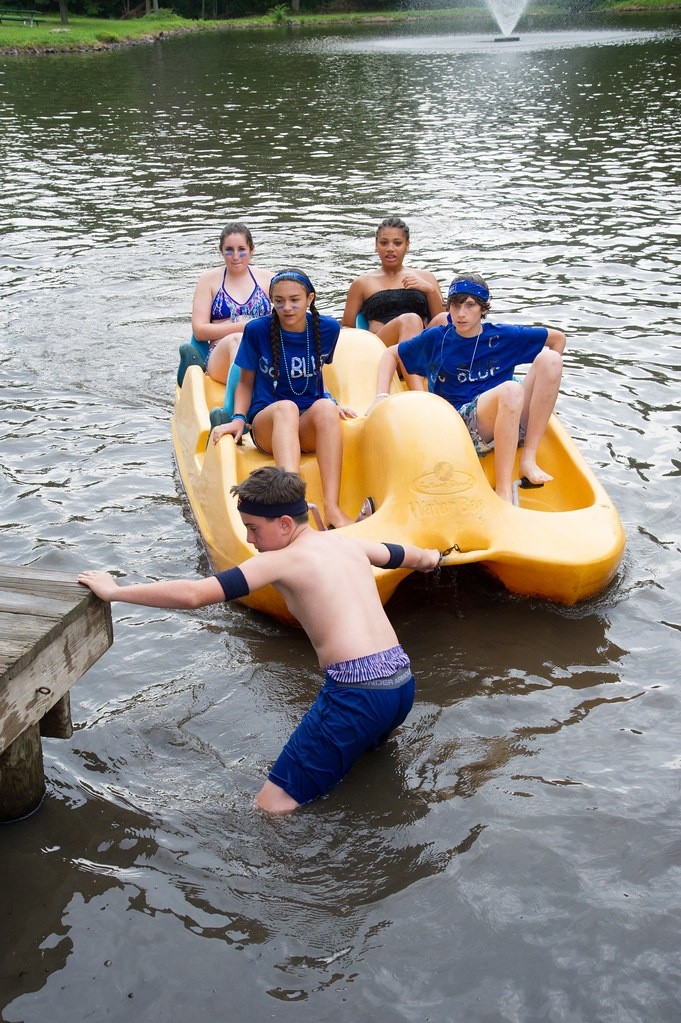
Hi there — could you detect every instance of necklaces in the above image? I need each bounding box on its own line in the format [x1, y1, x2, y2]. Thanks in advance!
[441, 325, 481, 377]
[279, 320, 310, 396]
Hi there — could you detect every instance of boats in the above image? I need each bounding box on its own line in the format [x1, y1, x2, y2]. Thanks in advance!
[169, 327, 627, 622]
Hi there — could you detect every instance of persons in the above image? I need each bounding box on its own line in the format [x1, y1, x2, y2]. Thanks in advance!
[340, 217, 450, 392]
[366, 273, 565, 507]
[212, 267, 357, 529]
[191, 223, 278, 383]
[76, 466, 438, 807]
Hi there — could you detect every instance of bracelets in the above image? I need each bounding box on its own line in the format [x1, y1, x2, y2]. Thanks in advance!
[324, 393, 331, 398]
[331, 398, 336, 402]
[376, 393, 389, 397]
[232, 414, 246, 421]
